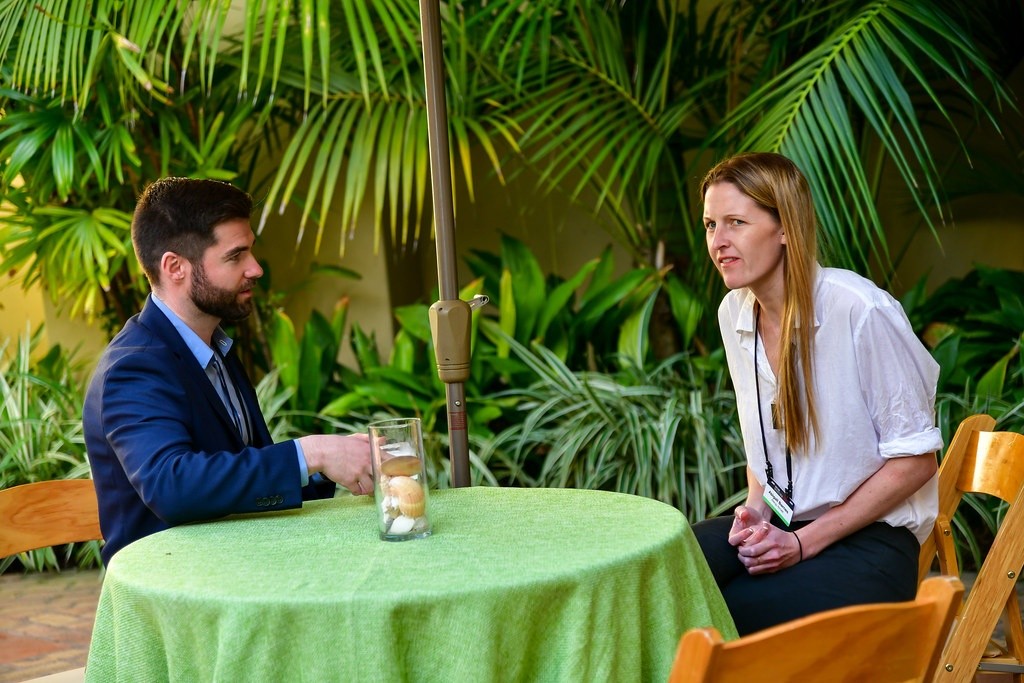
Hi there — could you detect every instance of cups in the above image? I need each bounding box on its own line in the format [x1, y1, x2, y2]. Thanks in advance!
[367, 417, 434, 543]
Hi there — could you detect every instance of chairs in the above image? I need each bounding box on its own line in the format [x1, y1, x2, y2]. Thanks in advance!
[918, 414, 1024, 683]
[0, 479, 104, 683]
[668, 576, 965, 683]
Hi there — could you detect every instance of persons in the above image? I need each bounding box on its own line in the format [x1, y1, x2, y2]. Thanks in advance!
[83, 177, 396, 570]
[691, 151, 944, 638]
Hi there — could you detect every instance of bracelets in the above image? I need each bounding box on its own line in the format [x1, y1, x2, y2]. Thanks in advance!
[792, 531, 803, 561]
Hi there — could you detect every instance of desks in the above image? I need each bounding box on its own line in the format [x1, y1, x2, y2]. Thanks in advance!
[87, 486, 741, 683]
